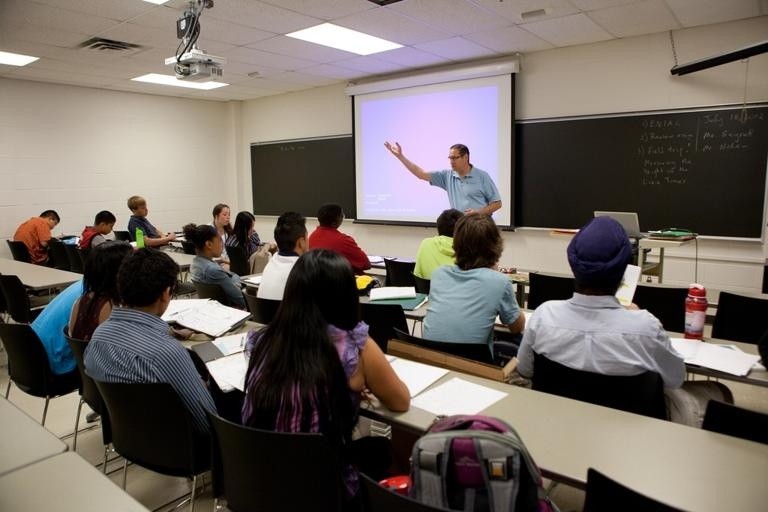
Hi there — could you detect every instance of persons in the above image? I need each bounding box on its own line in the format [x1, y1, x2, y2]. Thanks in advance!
[516, 216, 734, 429]
[383, 140, 502, 219]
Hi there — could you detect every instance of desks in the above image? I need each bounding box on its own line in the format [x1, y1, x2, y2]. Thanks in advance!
[627, 235, 696, 283]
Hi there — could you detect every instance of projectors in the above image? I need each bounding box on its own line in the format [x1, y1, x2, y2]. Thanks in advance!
[174, 61, 223, 83]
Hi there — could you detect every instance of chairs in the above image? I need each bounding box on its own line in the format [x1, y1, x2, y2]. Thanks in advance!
[0, 229, 768, 511]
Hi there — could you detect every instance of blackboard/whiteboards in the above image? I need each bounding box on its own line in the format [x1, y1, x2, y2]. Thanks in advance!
[249, 133, 355, 221]
[514, 101, 768, 244]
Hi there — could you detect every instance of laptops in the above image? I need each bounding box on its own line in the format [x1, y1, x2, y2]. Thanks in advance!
[593, 211, 647, 237]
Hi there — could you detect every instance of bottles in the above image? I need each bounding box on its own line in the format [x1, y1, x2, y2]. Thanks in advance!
[683, 284, 708, 342]
[135, 228, 145, 249]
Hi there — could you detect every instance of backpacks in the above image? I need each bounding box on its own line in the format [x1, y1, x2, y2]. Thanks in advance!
[410, 412, 562, 511]
